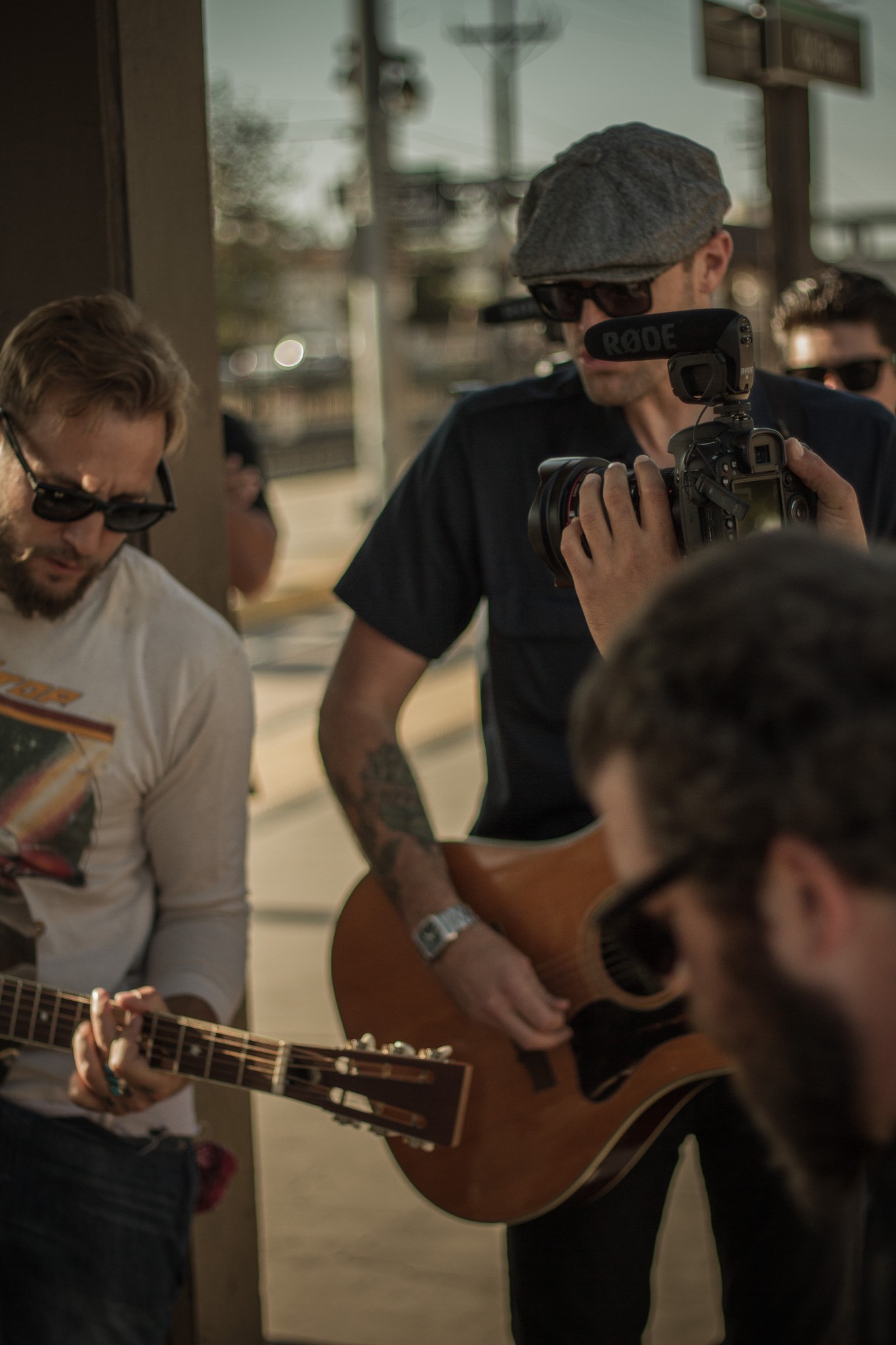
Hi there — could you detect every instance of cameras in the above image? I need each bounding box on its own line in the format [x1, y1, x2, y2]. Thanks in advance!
[525, 405, 815, 585]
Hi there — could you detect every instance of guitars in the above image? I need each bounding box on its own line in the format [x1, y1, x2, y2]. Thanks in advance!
[0, 877, 472, 1150]
[329, 816, 726, 1231]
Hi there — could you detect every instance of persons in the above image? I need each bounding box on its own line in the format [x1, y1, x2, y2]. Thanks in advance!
[558, 269, 896, 1345]
[318, 120, 896, 1345]
[0, 290, 257, 1345]
[213, 379, 283, 701]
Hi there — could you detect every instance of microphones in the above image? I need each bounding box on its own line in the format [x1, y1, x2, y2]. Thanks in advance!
[582, 307, 754, 363]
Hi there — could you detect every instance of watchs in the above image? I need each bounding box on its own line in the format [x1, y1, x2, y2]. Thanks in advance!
[406, 896, 478, 968]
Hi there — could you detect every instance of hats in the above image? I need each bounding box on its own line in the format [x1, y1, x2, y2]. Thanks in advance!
[512, 122, 731, 283]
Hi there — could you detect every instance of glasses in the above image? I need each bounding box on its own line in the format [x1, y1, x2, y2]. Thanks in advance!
[783, 357, 896, 394]
[595, 817, 863, 993]
[526, 272, 667, 324]
[0, 413, 177, 532]
